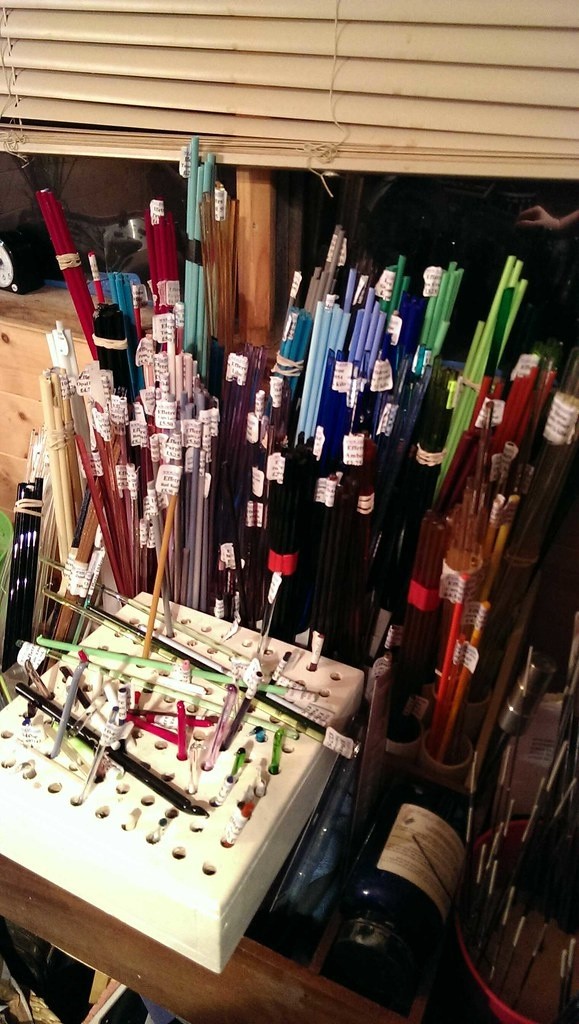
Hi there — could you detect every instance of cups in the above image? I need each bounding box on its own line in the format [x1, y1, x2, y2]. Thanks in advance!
[386, 715, 423, 752]
[422, 725, 474, 783]
[432, 674, 492, 737]
